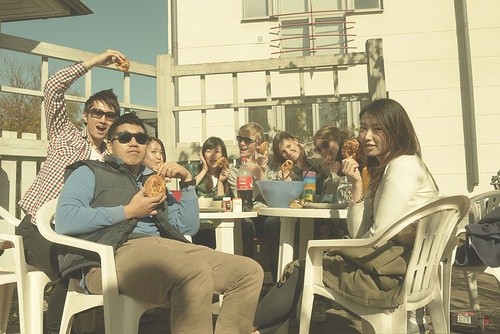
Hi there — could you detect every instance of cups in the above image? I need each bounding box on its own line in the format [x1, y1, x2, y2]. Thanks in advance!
[170, 190, 181, 202]
[233, 198, 242, 212]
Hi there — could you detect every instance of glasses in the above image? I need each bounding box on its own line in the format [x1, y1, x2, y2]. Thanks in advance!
[313, 138, 336, 154]
[236, 134, 256, 145]
[109, 131, 149, 144]
[86, 108, 119, 121]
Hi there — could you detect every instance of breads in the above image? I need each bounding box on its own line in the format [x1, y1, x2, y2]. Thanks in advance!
[281, 160, 293, 171]
[217, 156, 229, 168]
[115, 57, 130, 71]
[342, 138, 362, 159]
[258, 142, 270, 156]
[144, 175, 166, 197]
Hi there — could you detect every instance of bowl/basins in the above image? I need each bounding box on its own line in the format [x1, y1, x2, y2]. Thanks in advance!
[230, 187, 259, 201]
[255, 180, 305, 208]
[315, 194, 333, 202]
[198, 198, 214, 207]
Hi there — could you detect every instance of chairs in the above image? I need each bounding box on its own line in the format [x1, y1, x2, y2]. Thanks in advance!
[0, 205, 52, 333]
[441, 190, 500, 332]
[299, 195, 470, 334]
[35, 199, 192, 334]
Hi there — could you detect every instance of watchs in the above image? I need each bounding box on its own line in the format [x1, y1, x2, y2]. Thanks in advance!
[180, 179, 196, 188]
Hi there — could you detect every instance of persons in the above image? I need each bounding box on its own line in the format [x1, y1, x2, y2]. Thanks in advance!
[142, 137, 167, 177]
[301, 123, 377, 322]
[241, 98, 439, 334]
[179, 136, 230, 254]
[52, 111, 264, 334]
[263, 129, 337, 259]
[222, 121, 286, 286]
[16, 47, 137, 334]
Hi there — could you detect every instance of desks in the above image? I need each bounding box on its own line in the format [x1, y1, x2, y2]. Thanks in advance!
[257, 208, 348, 282]
[200, 211, 259, 308]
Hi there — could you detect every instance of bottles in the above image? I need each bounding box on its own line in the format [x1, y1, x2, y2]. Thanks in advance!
[337, 176, 352, 204]
[237, 157, 253, 212]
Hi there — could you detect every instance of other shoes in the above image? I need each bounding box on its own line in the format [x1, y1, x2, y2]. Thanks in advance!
[311, 296, 328, 324]
